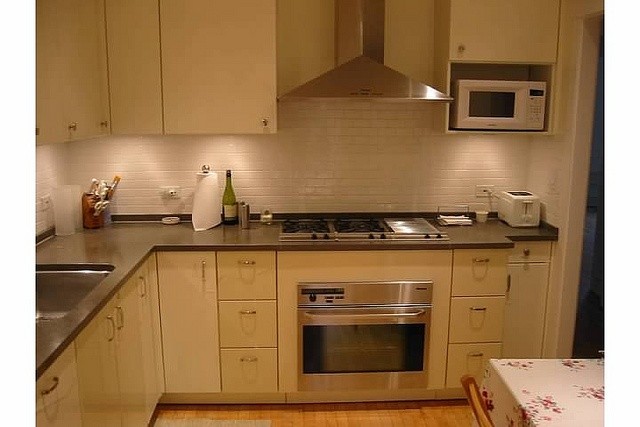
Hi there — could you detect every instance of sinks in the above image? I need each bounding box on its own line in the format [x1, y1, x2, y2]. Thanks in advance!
[36, 264, 115, 322]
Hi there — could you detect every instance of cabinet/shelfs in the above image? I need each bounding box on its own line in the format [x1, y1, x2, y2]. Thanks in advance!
[160, 2, 278, 135]
[507, 235, 552, 360]
[104, 0, 164, 138]
[446, 244, 507, 399]
[37, 0, 112, 143]
[217, 246, 277, 405]
[37, 338, 83, 427]
[155, 246, 221, 405]
[435, 0, 559, 137]
[138, 245, 165, 427]
[75, 251, 147, 427]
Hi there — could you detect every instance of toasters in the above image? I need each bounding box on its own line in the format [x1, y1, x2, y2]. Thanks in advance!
[497, 189, 541, 229]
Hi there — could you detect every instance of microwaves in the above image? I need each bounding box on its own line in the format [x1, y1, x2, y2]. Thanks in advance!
[451, 79, 548, 132]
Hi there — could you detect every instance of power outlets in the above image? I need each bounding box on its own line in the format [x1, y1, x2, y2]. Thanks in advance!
[474, 186, 495, 198]
[160, 186, 181, 200]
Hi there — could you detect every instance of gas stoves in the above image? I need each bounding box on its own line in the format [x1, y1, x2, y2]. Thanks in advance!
[279, 217, 444, 242]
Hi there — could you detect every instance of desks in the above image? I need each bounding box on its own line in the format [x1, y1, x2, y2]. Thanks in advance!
[480, 359, 604, 426]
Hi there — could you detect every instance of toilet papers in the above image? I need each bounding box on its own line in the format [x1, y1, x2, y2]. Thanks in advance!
[192, 173, 222, 232]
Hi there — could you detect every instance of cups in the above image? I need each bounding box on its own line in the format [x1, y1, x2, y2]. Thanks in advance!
[477, 210, 489, 224]
[260, 206, 275, 226]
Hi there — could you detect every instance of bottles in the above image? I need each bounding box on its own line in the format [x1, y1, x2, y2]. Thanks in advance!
[221, 169, 238, 227]
[239, 201, 249, 230]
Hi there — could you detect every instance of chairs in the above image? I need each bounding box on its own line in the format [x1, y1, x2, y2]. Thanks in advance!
[461, 374, 493, 426]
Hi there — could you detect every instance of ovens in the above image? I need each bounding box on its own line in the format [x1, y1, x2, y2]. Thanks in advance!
[297, 280, 433, 389]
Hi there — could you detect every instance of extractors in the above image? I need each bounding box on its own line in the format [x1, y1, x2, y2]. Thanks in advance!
[279, 54, 453, 105]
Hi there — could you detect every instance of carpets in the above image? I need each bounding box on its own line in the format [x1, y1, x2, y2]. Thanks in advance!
[155, 416, 272, 427]
